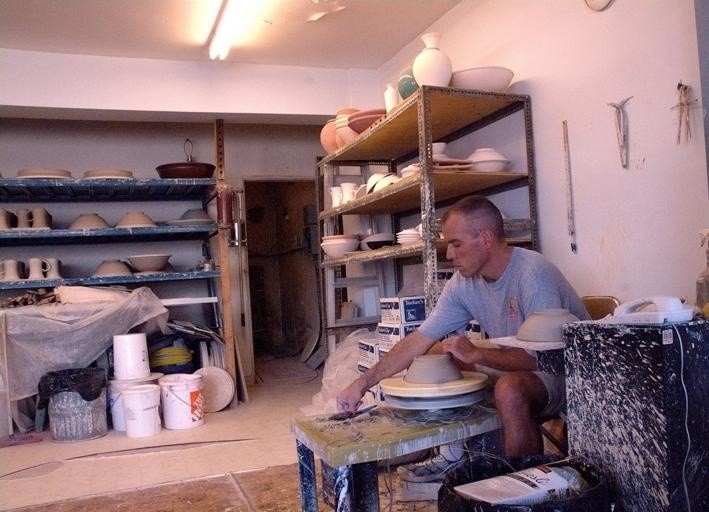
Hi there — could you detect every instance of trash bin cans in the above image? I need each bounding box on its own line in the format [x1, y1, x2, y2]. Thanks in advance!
[43, 367, 108, 443]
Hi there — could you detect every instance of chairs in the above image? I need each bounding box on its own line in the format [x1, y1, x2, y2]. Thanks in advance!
[541, 295, 621, 457]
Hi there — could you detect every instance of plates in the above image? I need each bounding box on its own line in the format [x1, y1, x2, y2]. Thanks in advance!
[166, 219, 215, 225]
[396, 230, 422, 244]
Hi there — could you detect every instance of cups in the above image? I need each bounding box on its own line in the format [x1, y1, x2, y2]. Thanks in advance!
[330, 182, 358, 206]
[0, 208, 48, 230]
[0, 258, 63, 281]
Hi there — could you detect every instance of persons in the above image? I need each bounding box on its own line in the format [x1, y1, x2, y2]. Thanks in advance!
[336, 194, 594, 457]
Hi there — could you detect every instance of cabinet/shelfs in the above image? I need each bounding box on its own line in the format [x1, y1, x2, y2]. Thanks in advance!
[314, 84, 539, 364]
[1, 118, 238, 409]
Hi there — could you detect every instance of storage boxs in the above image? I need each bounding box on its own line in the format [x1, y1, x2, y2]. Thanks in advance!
[356, 295, 426, 403]
[463, 318, 483, 342]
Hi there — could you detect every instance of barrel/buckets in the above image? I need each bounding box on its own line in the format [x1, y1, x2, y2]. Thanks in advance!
[158, 375, 206, 430]
[122, 384, 162, 438]
[109, 380, 153, 431]
[113, 333, 152, 380]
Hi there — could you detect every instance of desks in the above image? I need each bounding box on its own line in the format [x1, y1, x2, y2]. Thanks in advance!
[561, 320, 709, 512]
[291, 405, 504, 511]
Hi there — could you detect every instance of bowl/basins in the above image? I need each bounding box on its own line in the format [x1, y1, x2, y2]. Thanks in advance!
[366, 172, 400, 192]
[468, 148, 509, 172]
[115, 211, 154, 227]
[404, 355, 463, 384]
[430, 142, 447, 153]
[181, 209, 209, 219]
[155, 162, 216, 178]
[320, 232, 395, 256]
[70, 213, 110, 229]
[94, 259, 132, 275]
[127, 254, 172, 270]
[452, 66, 515, 91]
[516, 309, 581, 341]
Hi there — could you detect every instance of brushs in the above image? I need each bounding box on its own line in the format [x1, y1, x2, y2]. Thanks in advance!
[329, 404, 377, 421]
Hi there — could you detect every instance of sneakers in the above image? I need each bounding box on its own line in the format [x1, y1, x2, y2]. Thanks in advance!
[398, 453, 468, 482]
